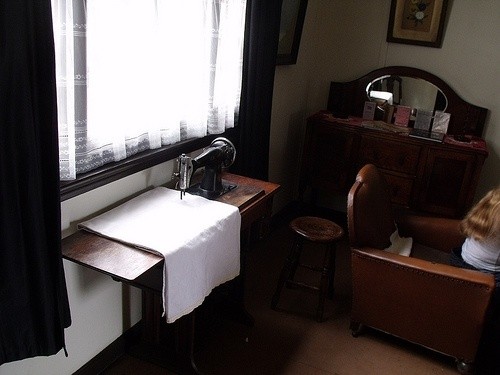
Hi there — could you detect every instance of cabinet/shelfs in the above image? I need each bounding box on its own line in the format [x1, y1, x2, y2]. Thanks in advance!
[297, 65, 488, 218]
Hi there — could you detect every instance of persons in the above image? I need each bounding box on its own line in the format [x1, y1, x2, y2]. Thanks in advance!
[450, 182, 500, 287]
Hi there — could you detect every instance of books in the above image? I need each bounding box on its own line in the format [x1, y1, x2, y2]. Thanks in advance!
[408, 128, 445, 143]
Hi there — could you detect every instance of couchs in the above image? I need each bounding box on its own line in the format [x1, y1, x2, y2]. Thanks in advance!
[346, 163, 496, 373]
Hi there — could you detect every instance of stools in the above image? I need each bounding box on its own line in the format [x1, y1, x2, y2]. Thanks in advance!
[268, 216, 345, 324]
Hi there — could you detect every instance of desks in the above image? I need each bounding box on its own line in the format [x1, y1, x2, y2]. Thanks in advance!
[61, 171, 281, 375]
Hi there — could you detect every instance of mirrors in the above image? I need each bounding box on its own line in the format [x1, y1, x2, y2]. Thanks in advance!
[365, 75, 449, 121]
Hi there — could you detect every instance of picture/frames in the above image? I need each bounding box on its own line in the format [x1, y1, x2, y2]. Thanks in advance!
[386, 0, 448, 48]
